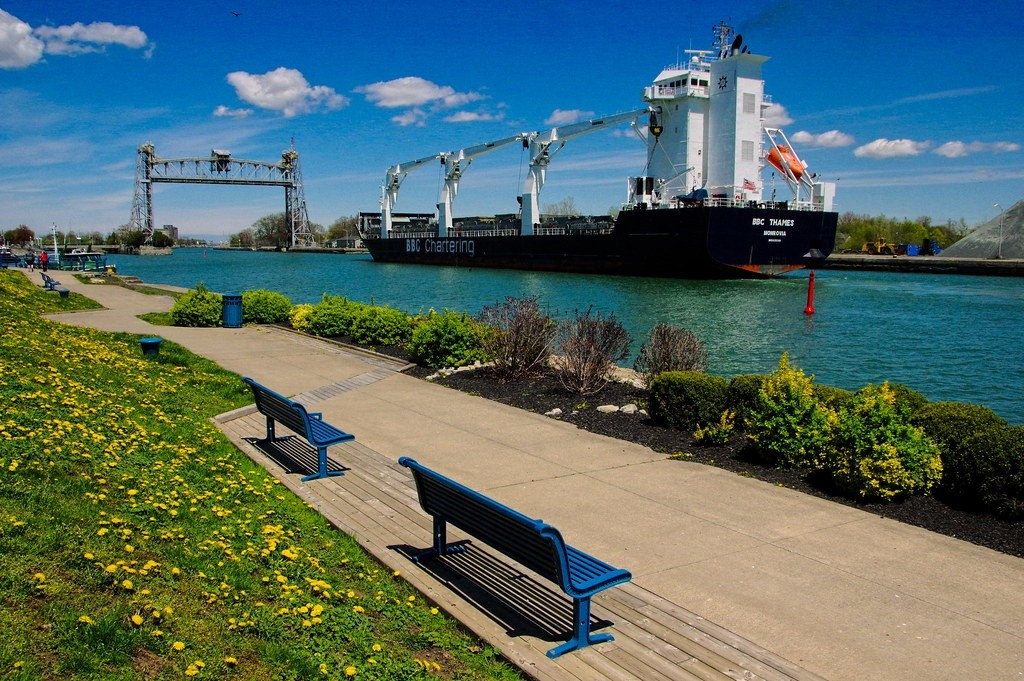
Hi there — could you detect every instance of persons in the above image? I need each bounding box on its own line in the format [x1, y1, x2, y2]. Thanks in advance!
[28, 252, 34, 272]
[41, 250, 48, 272]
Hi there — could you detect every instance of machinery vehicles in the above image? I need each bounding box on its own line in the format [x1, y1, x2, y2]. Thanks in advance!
[861, 238, 899, 254]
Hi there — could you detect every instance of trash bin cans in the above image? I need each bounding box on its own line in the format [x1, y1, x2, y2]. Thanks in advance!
[222, 290, 243, 328]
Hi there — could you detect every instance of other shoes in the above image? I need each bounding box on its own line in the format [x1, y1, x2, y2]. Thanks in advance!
[28, 270, 30, 272]
[32, 269, 34, 272]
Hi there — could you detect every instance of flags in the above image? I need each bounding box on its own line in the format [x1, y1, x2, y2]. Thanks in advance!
[743, 178, 756, 190]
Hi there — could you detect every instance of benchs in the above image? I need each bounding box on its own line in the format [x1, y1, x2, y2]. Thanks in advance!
[399, 455, 632, 658]
[242, 376, 355, 482]
[40, 271, 62, 291]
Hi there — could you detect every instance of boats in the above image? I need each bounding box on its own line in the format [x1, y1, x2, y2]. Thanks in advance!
[358, 18, 840, 276]
[62, 249, 101, 261]
